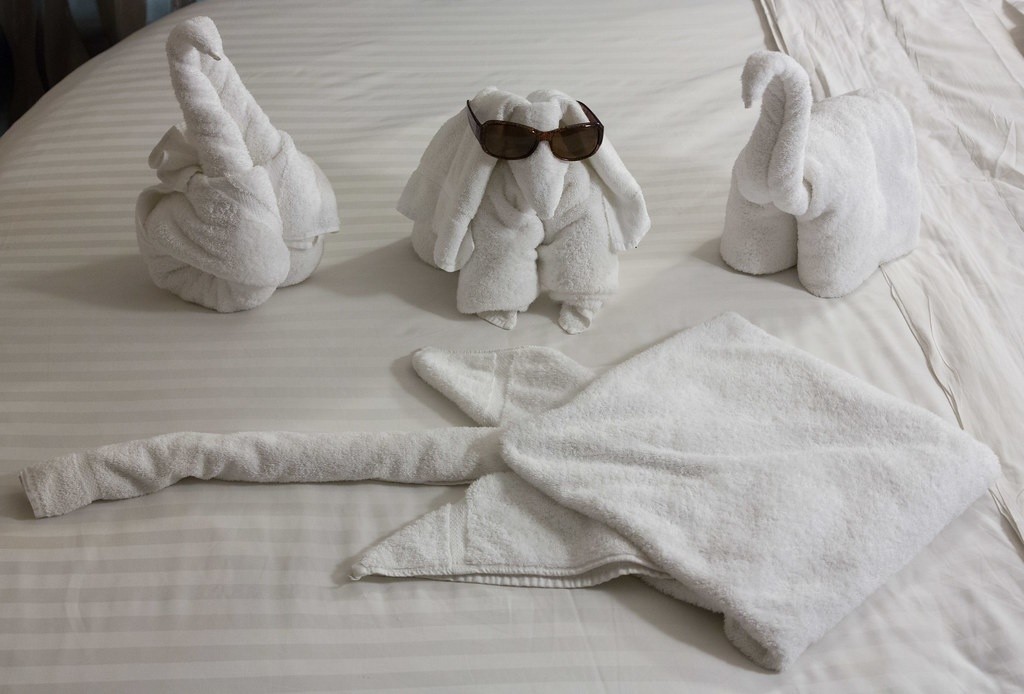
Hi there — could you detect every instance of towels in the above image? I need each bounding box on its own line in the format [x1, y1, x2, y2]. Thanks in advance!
[721, 45, 920, 298]
[134, 15, 341, 314]
[19, 307, 1005, 673]
[395, 85, 653, 333]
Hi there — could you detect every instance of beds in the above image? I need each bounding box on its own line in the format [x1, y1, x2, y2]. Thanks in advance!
[2, 0, 1022, 693]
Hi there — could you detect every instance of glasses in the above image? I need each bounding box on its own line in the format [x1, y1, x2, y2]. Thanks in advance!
[466, 99, 604, 162]
